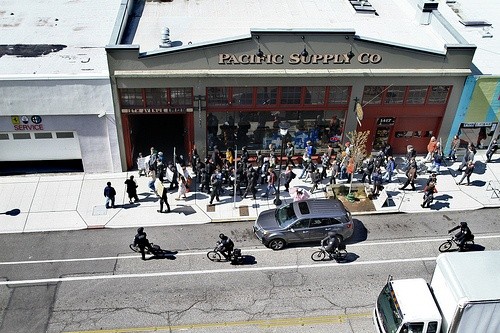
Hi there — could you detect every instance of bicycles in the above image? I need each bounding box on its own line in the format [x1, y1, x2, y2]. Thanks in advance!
[311, 242, 348, 262]
[207, 243, 242, 263]
[439, 232, 475, 252]
[129, 241, 165, 258]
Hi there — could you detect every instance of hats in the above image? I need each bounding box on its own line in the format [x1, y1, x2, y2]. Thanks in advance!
[219, 234, 224, 239]
[137, 227, 144, 231]
[328, 232, 336, 237]
[461, 222, 467, 227]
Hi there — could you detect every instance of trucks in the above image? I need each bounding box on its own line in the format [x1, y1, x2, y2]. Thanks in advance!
[372, 250, 500, 333]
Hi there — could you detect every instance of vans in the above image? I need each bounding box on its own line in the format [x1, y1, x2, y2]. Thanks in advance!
[252, 199, 354, 251]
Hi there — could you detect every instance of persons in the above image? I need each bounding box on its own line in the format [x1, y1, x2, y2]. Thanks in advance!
[157, 187, 170, 213]
[206, 112, 340, 143]
[448, 222, 471, 252]
[485, 139, 498, 163]
[124, 175, 139, 203]
[137, 135, 478, 208]
[132, 227, 152, 261]
[445, 134, 461, 160]
[104, 182, 116, 208]
[320, 232, 339, 259]
[455, 160, 474, 186]
[217, 233, 234, 261]
